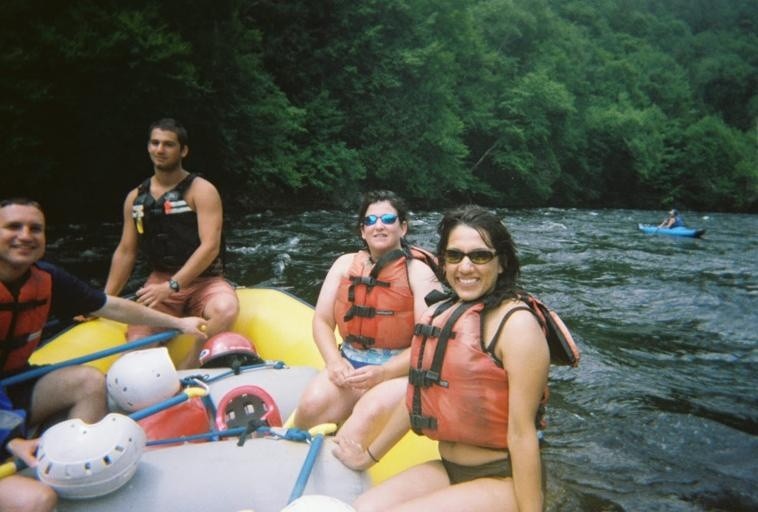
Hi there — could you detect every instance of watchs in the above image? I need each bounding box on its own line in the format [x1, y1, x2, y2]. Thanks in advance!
[167, 277, 180, 294]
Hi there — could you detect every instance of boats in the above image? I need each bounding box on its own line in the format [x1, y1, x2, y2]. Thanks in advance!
[1, 287, 444, 512]
[637, 222, 706, 238]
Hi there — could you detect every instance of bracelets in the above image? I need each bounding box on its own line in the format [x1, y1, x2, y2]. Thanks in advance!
[366, 446, 382, 463]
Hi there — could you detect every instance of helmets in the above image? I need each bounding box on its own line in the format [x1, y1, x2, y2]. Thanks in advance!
[212, 385, 285, 443]
[103, 346, 182, 413]
[35, 411, 147, 502]
[198, 330, 266, 367]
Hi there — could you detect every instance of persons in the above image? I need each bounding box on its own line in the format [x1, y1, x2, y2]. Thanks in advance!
[0, 199, 208, 418]
[71, 118, 241, 369]
[663, 209, 687, 230]
[292, 191, 444, 454]
[328, 204, 552, 512]
[0, 405, 59, 512]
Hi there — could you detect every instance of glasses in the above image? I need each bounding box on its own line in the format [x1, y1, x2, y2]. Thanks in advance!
[442, 248, 500, 265]
[360, 213, 402, 226]
